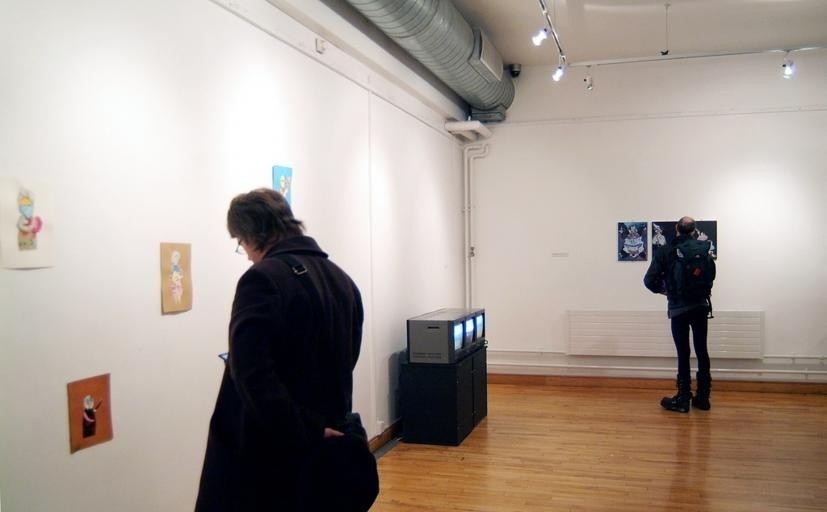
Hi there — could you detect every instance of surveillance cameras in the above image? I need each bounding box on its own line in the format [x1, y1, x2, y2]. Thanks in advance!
[509, 63, 521, 77]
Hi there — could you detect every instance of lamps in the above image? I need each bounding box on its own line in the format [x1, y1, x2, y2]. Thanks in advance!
[781, 50, 795, 80]
[533, 11, 596, 92]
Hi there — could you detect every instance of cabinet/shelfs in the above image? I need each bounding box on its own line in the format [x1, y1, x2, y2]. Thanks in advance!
[398, 346, 488, 447]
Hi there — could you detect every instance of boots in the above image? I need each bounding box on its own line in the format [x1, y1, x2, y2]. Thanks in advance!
[661, 388, 710, 413]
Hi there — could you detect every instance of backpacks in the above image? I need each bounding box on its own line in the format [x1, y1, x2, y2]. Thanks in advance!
[668, 236, 715, 303]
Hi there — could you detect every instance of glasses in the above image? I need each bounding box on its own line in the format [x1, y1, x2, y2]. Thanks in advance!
[235, 237, 247, 255]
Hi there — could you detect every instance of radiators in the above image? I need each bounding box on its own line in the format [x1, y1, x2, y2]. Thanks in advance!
[562, 311, 766, 361]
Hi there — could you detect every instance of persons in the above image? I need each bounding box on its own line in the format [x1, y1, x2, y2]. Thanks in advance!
[188, 184, 383, 511]
[640, 216, 716, 413]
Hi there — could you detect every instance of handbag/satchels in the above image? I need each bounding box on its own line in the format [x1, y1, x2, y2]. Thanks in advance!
[285, 422, 379, 512]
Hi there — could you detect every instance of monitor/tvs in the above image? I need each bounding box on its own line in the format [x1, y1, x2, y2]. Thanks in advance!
[407, 308, 484, 364]
